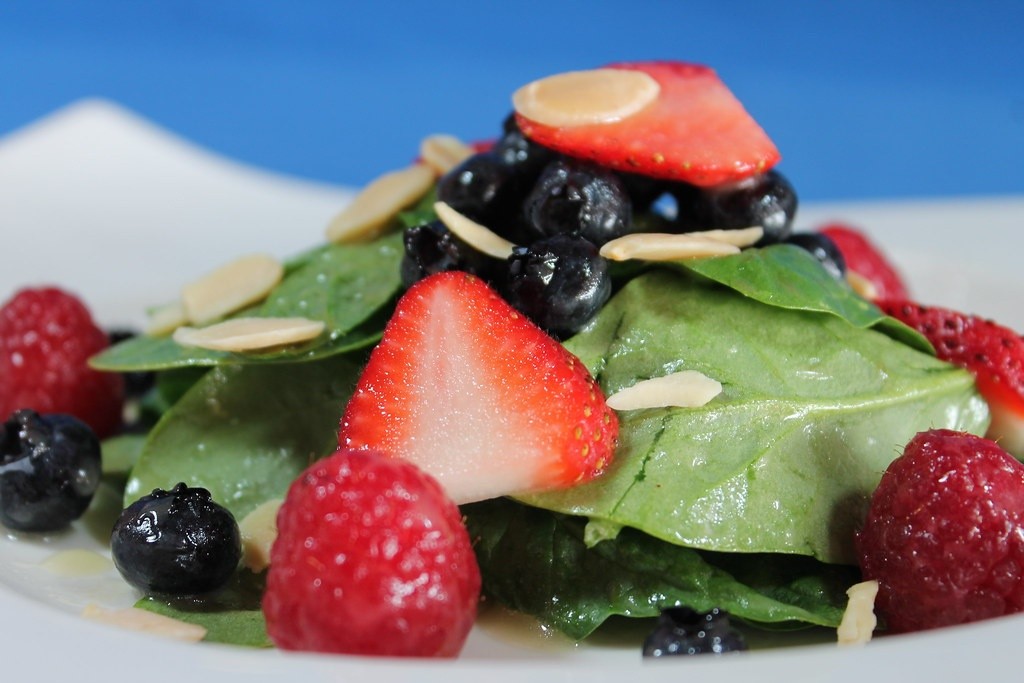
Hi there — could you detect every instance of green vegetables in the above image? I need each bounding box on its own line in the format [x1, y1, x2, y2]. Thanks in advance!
[81, 182, 990, 654]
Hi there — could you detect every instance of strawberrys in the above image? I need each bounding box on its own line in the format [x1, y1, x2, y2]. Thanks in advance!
[514, 60, 781, 186]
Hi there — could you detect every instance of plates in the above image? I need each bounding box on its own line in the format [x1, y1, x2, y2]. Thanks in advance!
[1, 93, 1020, 677]
